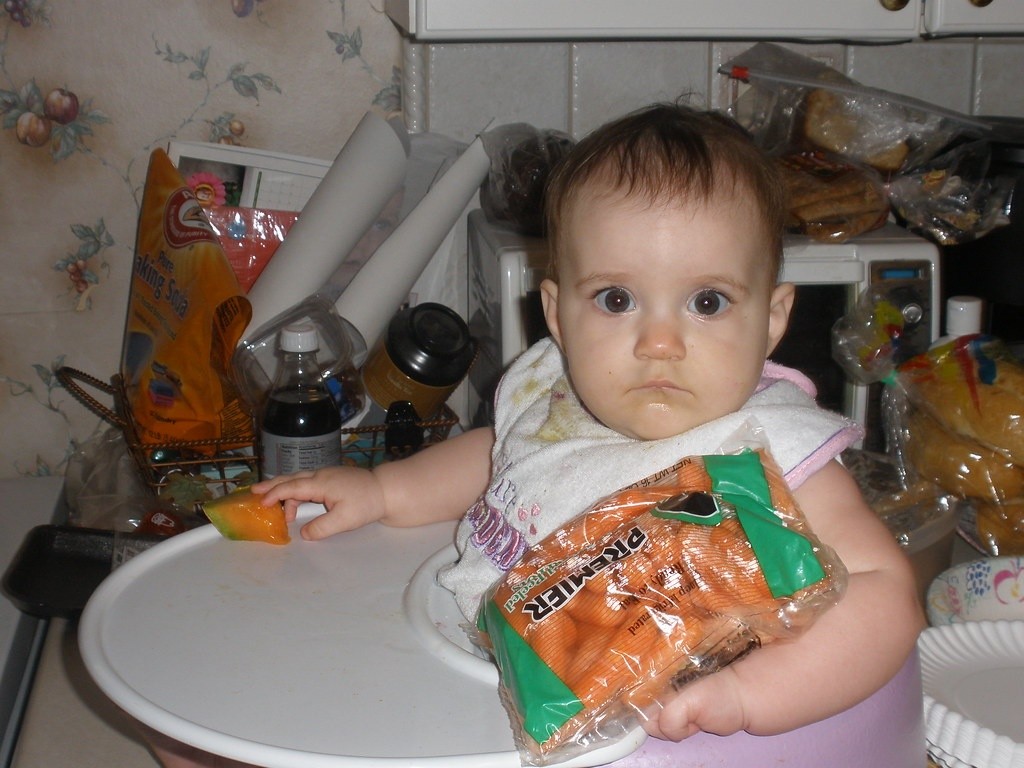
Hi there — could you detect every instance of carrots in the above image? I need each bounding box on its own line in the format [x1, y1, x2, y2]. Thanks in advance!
[475, 447, 848, 765]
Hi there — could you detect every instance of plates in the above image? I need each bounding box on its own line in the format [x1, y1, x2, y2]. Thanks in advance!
[917, 620, 1024, 768]
[925, 554, 1024, 628]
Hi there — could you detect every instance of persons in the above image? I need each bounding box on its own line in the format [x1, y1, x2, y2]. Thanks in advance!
[252, 102, 930, 745]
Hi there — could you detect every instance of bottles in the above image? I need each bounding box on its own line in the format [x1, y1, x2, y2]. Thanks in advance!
[258, 324, 342, 482]
[930, 296, 983, 354]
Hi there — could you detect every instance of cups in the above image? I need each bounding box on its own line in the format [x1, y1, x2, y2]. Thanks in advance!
[362, 302, 475, 434]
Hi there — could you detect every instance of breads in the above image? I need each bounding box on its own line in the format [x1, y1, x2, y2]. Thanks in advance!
[760, 86, 977, 246]
[890, 344, 1024, 559]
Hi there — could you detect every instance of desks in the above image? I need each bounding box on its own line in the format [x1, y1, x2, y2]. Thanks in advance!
[79, 503, 650, 768]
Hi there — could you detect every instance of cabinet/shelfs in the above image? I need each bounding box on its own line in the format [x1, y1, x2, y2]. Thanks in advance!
[382, 0, 1024, 43]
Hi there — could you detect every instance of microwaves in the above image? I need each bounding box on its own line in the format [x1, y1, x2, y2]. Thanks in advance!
[464, 208, 941, 467]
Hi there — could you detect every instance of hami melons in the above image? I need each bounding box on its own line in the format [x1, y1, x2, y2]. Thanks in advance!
[201, 485, 292, 545]
